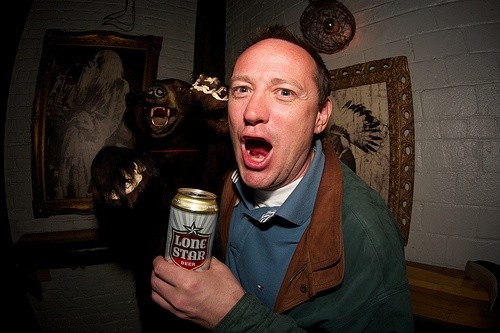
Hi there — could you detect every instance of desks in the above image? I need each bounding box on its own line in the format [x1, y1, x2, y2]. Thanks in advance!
[404, 261, 500, 333]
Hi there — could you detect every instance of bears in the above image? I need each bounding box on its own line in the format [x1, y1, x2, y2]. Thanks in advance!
[88, 80, 238, 333]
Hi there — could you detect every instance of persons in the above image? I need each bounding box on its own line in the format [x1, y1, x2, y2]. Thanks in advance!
[147, 25, 407, 333]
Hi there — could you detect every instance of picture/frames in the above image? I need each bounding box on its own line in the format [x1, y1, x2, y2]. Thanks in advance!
[325, 57, 415, 246]
[29, 29, 163, 215]
